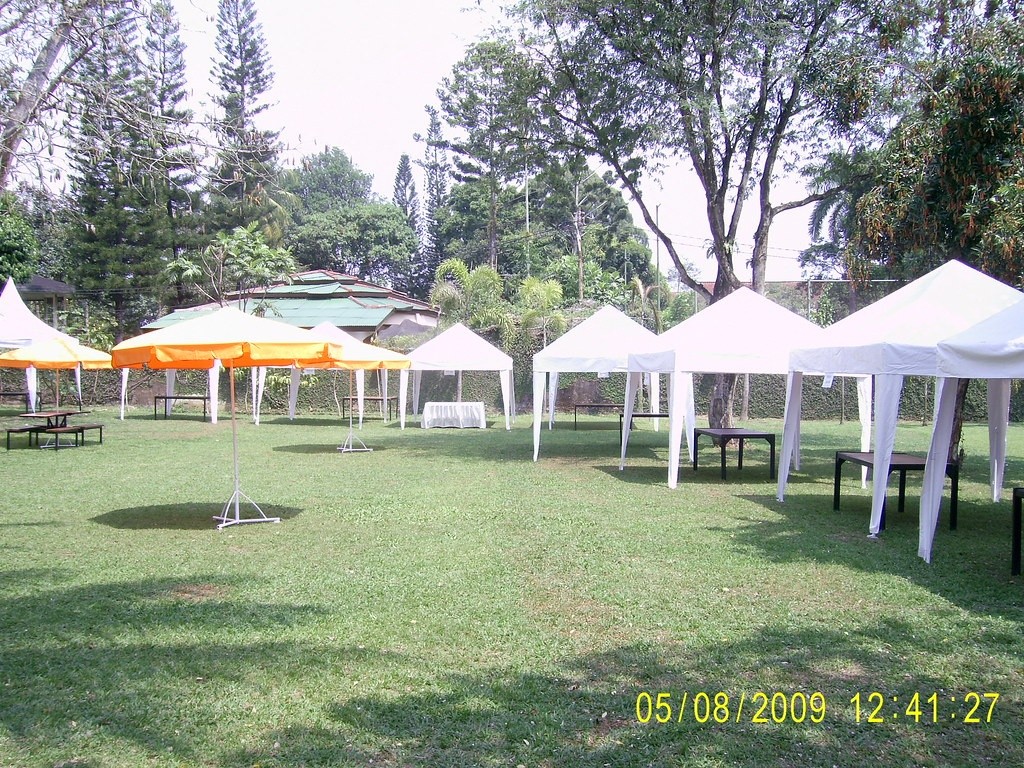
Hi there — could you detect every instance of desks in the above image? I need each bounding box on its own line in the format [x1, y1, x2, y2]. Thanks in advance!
[153, 394, 210, 422]
[1010, 487, 1024, 575]
[420, 401, 487, 429]
[342, 396, 398, 421]
[694, 428, 775, 481]
[619, 413, 669, 445]
[574, 404, 633, 431]
[833, 451, 959, 531]
[0, 391, 42, 413]
[4, 410, 104, 451]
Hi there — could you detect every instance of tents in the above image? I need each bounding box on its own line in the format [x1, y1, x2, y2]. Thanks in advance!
[532, 304, 697, 463]
[399, 323, 516, 430]
[619, 286, 871, 489]
[120, 314, 296, 426]
[776, 258, 1024, 563]
[0, 276, 82, 413]
[255, 322, 387, 429]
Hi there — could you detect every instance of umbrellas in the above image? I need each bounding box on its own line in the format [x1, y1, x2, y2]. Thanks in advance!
[0, 338, 113, 412]
[111, 307, 342, 529]
[298, 341, 410, 428]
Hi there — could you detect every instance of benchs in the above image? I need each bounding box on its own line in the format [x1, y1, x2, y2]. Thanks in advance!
[6, 424, 104, 433]
